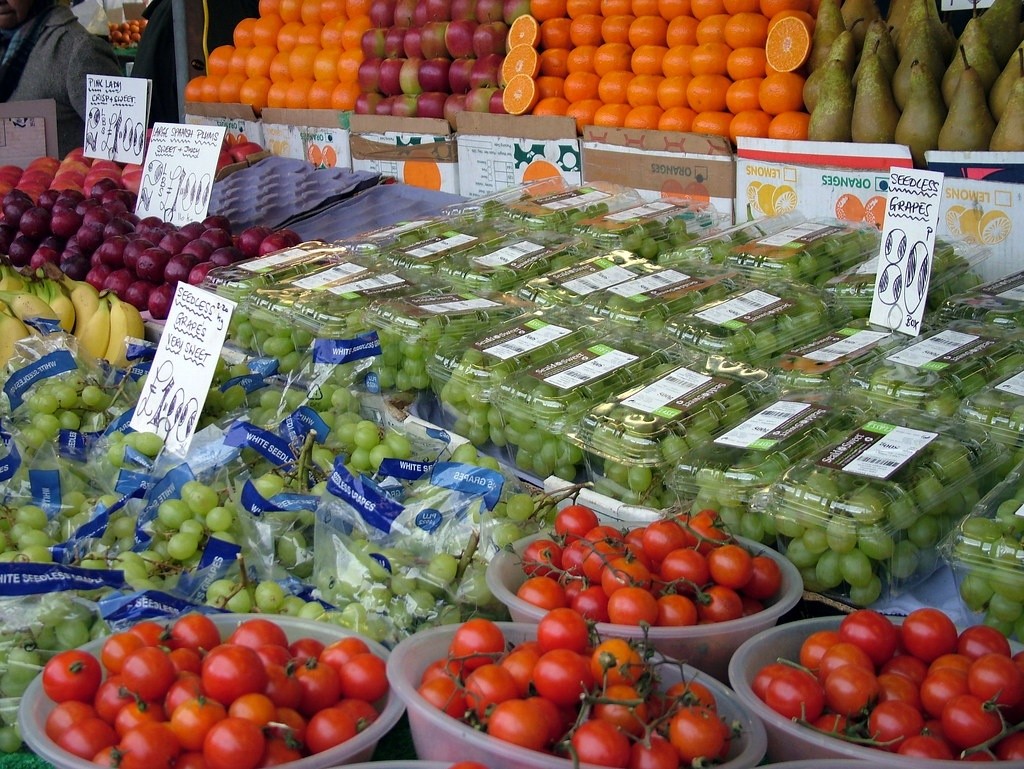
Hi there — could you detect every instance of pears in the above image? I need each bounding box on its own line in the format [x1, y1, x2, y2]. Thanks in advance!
[802, 0, 1024, 151]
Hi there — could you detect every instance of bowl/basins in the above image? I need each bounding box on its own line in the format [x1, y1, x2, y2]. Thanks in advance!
[18, 613, 406, 769]
[728, 616, 1024, 767]
[486, 525, 803, 683]
[384, 619, 768, 769]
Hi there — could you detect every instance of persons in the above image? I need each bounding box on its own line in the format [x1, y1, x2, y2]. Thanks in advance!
[1, 0, 127, 162]
[130, 0, 263, 131]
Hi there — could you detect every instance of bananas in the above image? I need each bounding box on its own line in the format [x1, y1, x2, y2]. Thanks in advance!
[0, 247, 145, 369]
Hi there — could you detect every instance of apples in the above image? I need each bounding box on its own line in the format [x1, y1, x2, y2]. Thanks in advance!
[0, 128, 264, 213]
[355, 0, 533, 130]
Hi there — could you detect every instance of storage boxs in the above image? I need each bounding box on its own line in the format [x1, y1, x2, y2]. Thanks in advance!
[349, 114, 458, 199]
[260, 107, 352, 173]
[0, 174, 1024, 769]
[581, 124, 736, 225]
[737, 136, 914, 233]
[184, 102, 263, 148]
[924, 151, 1024, 283]
[455, 110, 581, 199]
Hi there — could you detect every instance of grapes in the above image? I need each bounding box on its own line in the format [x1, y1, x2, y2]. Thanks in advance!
[0, 182, 1024, 748]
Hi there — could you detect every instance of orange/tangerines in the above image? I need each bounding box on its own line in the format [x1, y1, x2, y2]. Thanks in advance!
[111, 20, 148, 46]
[503, 0, 812, 140]
[185, 0, 372, 111]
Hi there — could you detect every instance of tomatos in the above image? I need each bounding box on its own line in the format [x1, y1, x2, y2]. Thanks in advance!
[45, 505, 1024, 769]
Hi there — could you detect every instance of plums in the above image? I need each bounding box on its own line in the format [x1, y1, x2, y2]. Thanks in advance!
[0, 178, 328, 320]
